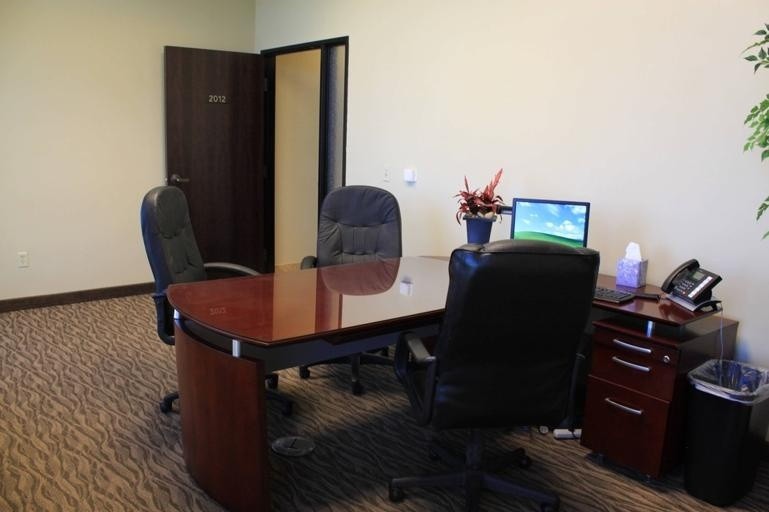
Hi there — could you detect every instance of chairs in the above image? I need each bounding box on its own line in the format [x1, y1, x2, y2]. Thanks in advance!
[300, 185, 403, 396]
[141, 184, 296, 419]
[384, 239, 600, 511]
[317, 259, 399, 298]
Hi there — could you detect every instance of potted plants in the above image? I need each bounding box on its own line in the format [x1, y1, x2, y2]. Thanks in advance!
[454, 169, 506, 243]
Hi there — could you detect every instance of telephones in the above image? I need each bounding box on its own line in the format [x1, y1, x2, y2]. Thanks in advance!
[660, 257, 723, 313]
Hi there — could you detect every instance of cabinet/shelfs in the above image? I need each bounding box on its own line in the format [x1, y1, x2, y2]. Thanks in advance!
[590, 307, 741, 489]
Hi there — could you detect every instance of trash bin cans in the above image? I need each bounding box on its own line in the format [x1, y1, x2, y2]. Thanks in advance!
[681, 358, 769, 508]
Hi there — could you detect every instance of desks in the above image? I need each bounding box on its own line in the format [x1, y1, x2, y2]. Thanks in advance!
[166, 243, 724, 512]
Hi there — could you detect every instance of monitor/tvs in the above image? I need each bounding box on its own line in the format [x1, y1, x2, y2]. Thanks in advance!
[510, 198, 590, 247]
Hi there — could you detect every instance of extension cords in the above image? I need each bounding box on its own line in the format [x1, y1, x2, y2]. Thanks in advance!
[553, 429, 583, 439]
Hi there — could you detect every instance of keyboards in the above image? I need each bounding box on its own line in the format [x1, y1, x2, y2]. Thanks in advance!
[593, 287, 634, 304]
